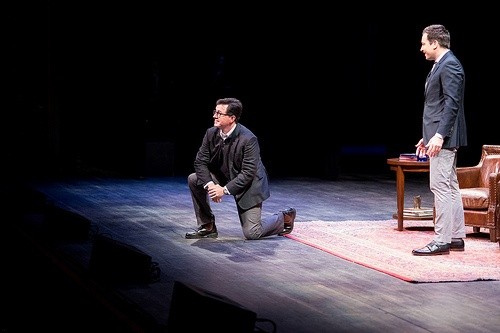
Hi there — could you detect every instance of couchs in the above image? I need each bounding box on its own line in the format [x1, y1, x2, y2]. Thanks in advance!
[454, 145, 500, 242]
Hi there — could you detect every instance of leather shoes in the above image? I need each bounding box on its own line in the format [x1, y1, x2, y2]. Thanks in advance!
[413, 240, 451, 255]
[449, 239, 465, 251]
[185, 225, 218, 238]
[278, 207, 297, 236]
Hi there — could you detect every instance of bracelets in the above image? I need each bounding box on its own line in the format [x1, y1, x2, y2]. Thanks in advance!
[223, 189, 229, 195]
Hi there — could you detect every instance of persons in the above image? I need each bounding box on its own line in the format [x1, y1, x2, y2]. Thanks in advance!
[185, 98, 297, 240]
[412, 24, 467, 256]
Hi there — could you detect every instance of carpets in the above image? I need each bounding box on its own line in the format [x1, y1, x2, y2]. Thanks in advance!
[279, 218, 500, 283]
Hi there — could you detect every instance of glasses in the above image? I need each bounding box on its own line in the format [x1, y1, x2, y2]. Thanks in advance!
[213, 110, 231, 116]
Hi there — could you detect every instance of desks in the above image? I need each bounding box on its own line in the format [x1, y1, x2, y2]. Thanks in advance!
[387, 158, 435, 231]
